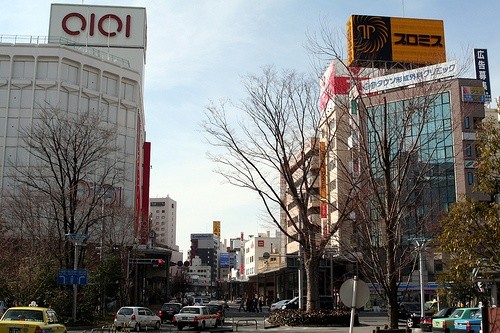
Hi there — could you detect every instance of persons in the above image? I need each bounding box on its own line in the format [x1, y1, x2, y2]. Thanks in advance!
[223, 299, 229, 311]
[187, 301, 192, 306]
[267, 298, 273, 311]
[239, 298, 262, 313]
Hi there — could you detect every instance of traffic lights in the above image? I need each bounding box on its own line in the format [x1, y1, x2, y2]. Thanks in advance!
[151, 259, 163, 267]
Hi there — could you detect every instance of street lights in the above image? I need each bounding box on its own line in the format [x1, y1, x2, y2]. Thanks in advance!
[407, 237, 434, 317]
[63, 233, 88, 323]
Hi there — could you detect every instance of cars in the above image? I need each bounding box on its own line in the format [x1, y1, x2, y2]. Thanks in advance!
[236, 297, 243, 303]
[270, 300, 290, 312]
[398, 301, 435, 328]
[0, 301, 69, 333]
[157, 295, 229, 331]
[424, 300, 482, 333]
[114, 306, 161, 332]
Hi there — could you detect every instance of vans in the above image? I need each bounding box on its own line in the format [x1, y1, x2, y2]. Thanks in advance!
[280, 295, 334, 311]
